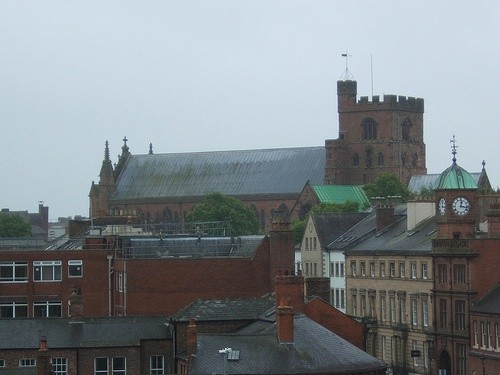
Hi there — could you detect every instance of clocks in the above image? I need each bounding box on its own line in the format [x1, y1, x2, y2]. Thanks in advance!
[452, 195, 471, 216]
[439, 198, 446, 216]
